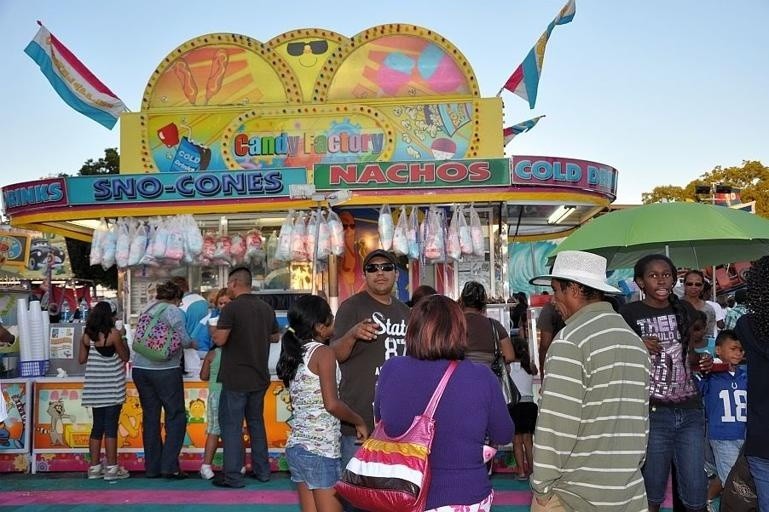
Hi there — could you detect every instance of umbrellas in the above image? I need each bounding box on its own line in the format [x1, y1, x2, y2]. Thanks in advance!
[544, 202, 769, 270]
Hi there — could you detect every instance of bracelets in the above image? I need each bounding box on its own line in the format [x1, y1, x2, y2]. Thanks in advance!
[121, 336, 127, 340]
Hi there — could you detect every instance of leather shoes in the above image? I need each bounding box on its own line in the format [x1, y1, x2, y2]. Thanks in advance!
[212, 480, 245, 488]
[166, 470, 191, 480]
[246, 469, 270, 482]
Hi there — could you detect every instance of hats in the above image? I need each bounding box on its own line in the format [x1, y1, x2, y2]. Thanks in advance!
[363, 249, 397, 271]
[529, 250, 623, 294]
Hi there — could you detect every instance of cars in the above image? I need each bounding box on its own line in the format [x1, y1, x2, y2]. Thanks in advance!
[28, 239, 65, 270]
[89, 295, 116, 315]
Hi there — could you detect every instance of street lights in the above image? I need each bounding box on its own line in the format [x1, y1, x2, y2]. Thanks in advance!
[695, 181, 731, 307]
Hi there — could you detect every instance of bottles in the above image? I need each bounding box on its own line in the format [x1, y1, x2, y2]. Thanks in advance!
[79, 297, 89, 323]
[59, 298, 71, 325]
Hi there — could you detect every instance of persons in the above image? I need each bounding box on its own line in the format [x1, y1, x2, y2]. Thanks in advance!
[611, 251, 768, 512]
[528, 251, 651, 512]
[275, 251, 517, 512]
[192, 267, 281, 487]
[131, 279, 192, 481]
[504, 290, 564, 480]
[79, 298, 131, 481]
[172, 276, 213, 350]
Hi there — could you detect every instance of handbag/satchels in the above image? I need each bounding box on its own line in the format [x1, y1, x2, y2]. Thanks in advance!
[491, 356, 521, 407]
[334, 416, 435, 512]
[132, 304, 184, 362]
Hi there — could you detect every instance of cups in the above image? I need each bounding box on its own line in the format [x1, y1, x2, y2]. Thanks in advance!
[209, 318, 217, 327]
[115, 320, 123, 331]
[16, 297, 50, 375]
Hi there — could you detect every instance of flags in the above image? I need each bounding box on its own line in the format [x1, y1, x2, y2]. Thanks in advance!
[503, 115, 541, 148]
[503, 0, 575, 109]
[23, 25, 124, 131]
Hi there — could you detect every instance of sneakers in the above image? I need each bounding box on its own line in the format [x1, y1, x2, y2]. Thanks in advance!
[88, 465, 104, 478]
[200, 464, 215, 479]
[104, 465, 130, 481]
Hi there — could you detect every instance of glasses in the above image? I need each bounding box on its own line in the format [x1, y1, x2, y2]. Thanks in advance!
[366, 262, 393, 273]
[686, 282, 703, 286]
